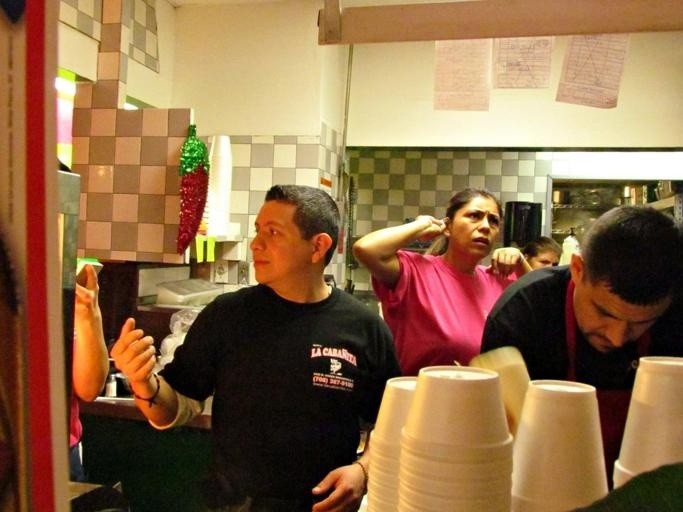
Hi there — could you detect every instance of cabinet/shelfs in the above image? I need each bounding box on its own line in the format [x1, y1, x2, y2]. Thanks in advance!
[546, 174, 683, 254]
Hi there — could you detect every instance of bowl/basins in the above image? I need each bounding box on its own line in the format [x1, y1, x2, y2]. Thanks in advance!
[394, 365, 514, 512]
[367, 373, 419, 512]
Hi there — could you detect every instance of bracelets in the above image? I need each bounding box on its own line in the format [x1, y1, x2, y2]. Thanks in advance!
[130, 372, 160, 407]
[519, 253, 525, 264]
[352, 461, 368, 495]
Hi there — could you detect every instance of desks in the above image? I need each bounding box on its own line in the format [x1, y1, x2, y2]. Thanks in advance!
[74, 394, 212, 431]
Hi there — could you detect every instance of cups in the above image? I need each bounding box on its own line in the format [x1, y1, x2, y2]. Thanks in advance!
[510, 379, 608, 511]
[612, 355, 683, 492]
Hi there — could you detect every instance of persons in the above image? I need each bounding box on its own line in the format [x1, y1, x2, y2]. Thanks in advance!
[110, 184, 403, 511]
[522, 237, 563, 272]
[0, 232, 26, 512]
[354, 187, 533, 378]
[57, 160, 109, 483]
[479, 206, 683, 493]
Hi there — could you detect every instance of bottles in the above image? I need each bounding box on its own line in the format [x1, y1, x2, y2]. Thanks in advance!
[105, 373, 118, 398]
